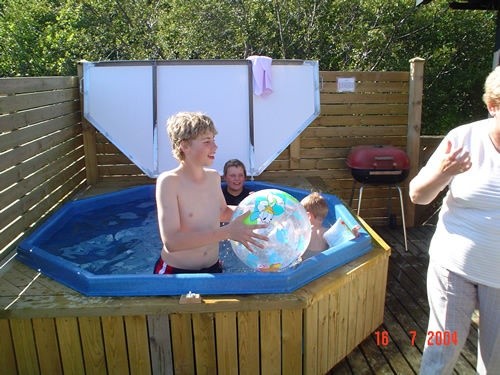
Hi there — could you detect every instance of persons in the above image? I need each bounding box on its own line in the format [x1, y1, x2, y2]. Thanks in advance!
[301, 192, 361, 252]
[154, 112, 269, 275]
[409, 65, 500, 375]
[223, 158, 255, 205]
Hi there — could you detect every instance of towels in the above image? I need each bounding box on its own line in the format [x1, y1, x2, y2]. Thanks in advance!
[246, 54, 274, 97]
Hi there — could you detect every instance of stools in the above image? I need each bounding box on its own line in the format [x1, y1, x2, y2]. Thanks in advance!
[349, 179, 408, 252]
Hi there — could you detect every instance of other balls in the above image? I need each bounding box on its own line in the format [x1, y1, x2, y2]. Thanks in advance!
[229, 189, 311, 272]
